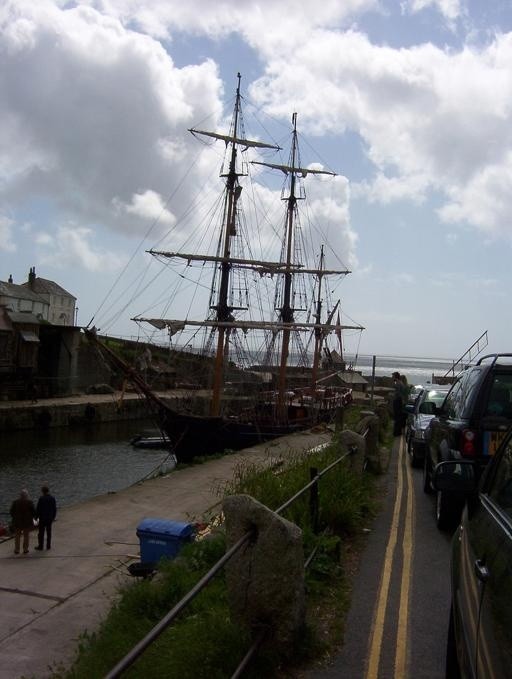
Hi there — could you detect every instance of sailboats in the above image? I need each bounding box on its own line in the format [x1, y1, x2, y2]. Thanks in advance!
[82, 69, 366, 469]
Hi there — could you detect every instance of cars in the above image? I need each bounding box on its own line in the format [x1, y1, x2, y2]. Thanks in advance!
[403, 351, 511, 678]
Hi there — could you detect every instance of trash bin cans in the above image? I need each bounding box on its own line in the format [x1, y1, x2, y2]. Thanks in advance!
[136, 518, 192, 564]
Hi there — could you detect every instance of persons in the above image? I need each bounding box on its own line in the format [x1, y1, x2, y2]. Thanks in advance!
[401, 376, 409, 427]
[388, 372, 405, 439]
[10, 489, 39, 555]
[34, 486, 58, 553]
